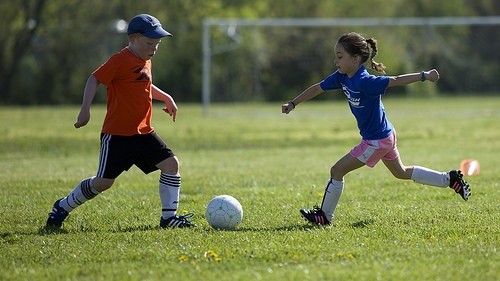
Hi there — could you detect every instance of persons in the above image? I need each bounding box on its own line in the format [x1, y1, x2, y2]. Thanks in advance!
[282, 33, 470, 226]
[46, 14, 198, 229]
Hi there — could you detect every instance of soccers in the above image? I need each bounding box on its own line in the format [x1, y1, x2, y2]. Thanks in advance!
[206, 195, 243, 230]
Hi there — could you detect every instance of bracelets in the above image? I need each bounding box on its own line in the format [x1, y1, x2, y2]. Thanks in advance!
[288, 101, 295, 108]
[420, 71, 425, 82]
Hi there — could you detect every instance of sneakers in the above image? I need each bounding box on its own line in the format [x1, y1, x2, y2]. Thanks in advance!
[47, 196, 69, 231]
[159, 213, 197, 230]
[300, 206, 331, 227]
[449, 170, 471, 201]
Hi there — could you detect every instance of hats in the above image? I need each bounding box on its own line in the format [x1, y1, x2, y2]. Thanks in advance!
[128, 14, 173, 39]
